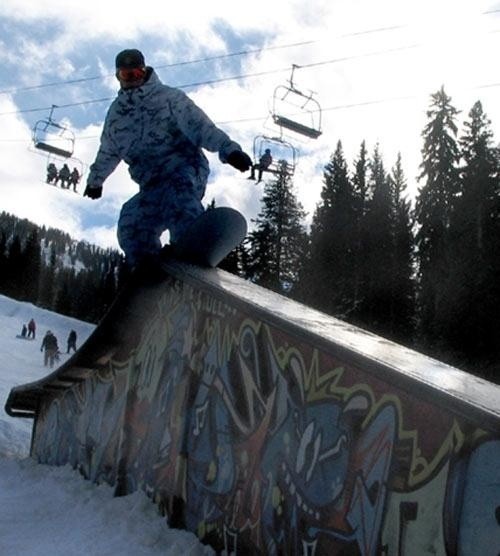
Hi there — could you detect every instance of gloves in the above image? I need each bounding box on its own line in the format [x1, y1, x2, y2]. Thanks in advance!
[227, 150, 252, 173]
[83, 185, 102, 200]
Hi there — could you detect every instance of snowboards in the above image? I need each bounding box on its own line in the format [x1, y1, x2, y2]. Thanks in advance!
[159, 208, 247, 269]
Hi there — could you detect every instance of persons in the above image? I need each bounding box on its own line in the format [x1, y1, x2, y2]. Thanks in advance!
[55, 347, 65, 359]
[46, 164, 57, 185]
[247, 147, 271, 185]
[21, 324, 28, 336]
[59, 163, 70, 188]
[67, 168, 79, 192]
[28, 318, 36, 339]
[83, 47, 253, 260]
[41, 330, 58, 368]
[66, 328, 77, 353]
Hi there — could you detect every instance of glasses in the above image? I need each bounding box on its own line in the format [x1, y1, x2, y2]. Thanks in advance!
[118, 66, 145, 82]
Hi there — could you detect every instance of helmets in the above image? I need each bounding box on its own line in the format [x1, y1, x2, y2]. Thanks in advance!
[115, 49, 145, 78]
